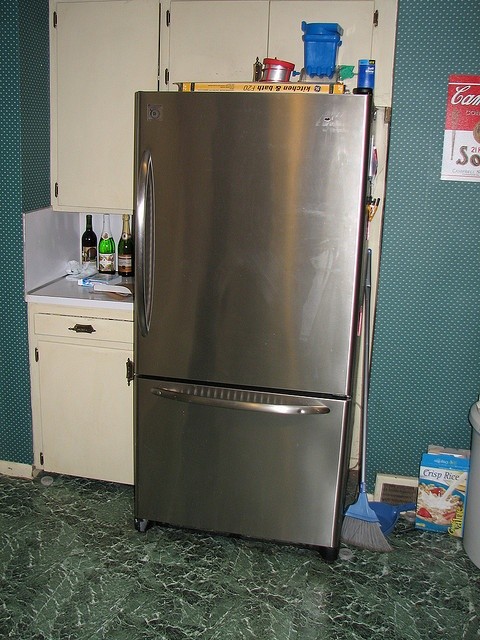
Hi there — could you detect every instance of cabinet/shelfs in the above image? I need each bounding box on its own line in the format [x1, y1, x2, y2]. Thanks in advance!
[166, 3, 374, 92]
[25, 304, 136, 487]
[54, 4, 157, 213]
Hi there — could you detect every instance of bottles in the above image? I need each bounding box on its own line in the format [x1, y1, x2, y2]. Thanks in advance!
[99, 213, 116, 275]
[82, 214, 97, 270]
[118, 214, 133, 277]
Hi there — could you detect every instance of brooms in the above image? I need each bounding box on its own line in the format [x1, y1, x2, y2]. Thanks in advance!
[341, 248, 393, 553]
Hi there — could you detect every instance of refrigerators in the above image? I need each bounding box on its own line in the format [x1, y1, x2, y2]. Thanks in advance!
[133, 90, 376, 562]
